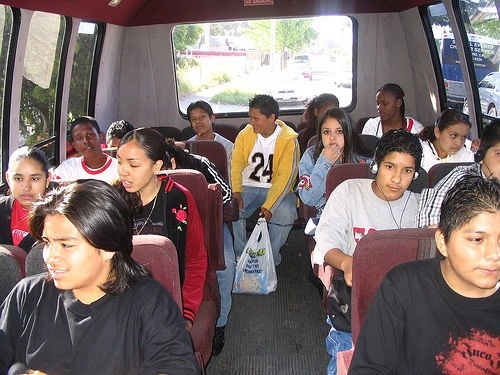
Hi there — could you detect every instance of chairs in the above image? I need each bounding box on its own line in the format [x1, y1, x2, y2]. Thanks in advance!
[0, 116, 500, 375]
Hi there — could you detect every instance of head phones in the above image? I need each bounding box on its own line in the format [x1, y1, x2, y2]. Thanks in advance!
[369, 130, 420, 181]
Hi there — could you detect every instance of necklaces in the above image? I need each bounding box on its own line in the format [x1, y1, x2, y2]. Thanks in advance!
[133, 185, 158, 236]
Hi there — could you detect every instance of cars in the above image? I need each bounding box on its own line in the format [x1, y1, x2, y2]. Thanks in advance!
[271, 53, 352, 107]
[462, 72, 500, 118]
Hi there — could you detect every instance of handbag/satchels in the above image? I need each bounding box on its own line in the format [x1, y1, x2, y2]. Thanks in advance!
[231, 216, 278, 295]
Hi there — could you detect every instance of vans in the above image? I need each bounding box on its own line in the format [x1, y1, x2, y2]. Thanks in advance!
[435, 32, 500, 110]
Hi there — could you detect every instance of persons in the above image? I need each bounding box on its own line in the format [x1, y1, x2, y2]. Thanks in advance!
[297, 108, 373, 217]
[297, 93, 340, 156]
[362, 83, 425, 138]
[0, 179, 200, 375]
[110, 128, 208, 331]
[310, 128, 422, 375]
[418, 118, 500, 256]
[54, 116, 121, 186]
[0, 146, 58, 256]
[187, 101, 234, 187]
[417, 109, 476, 173]
[231, 95, 297, 276]
[182, 154, 238, 356]
[347, 177, 500, 375]
[106, 119, 134, 149]
